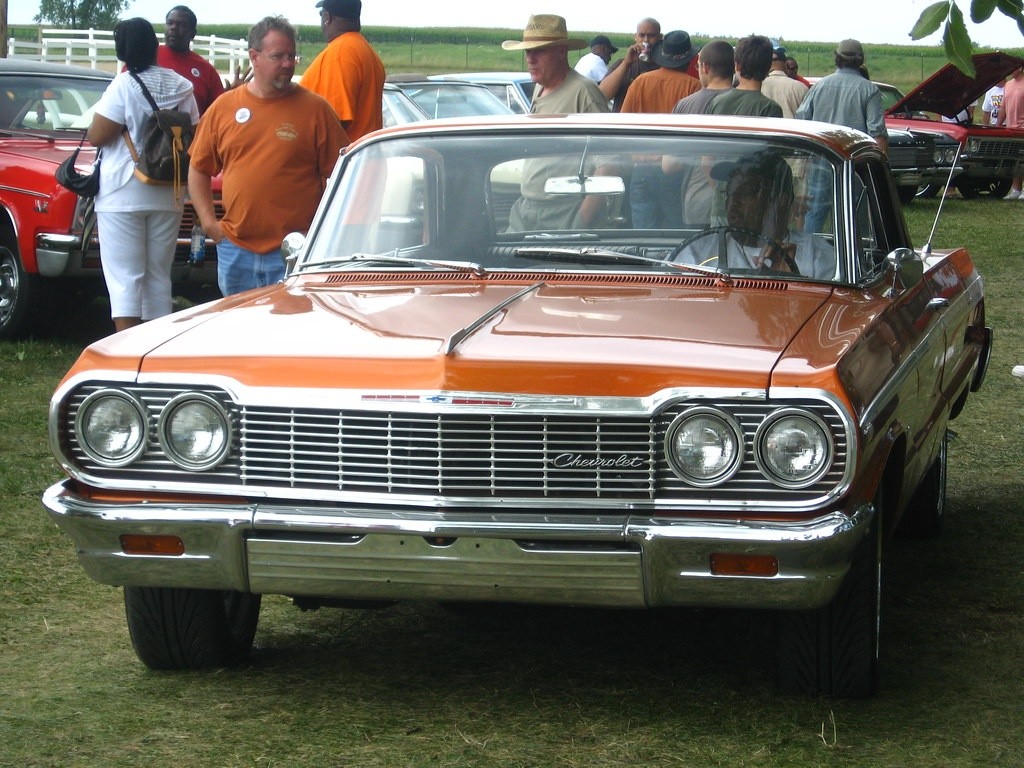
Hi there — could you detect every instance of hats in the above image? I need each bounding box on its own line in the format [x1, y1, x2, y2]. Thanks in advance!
[837, 38, 862, 59]
[501, 14, 587, 51]
[315, 0, 361, 17]
[709, 152, 794, 196]
[650, 30, 702, 68]
[591, 35, 618, 54]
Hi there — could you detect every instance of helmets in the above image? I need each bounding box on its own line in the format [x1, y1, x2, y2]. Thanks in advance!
[771, 39, 786, 53]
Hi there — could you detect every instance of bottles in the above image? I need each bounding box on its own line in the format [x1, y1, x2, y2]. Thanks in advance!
[188, 218, 205, 267]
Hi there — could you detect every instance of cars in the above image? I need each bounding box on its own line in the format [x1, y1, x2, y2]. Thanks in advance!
[38, 110, 997, 709]
[800, 50, 1024, 206]
[289, 70, 541, 259]
[0, 55, 223, 344]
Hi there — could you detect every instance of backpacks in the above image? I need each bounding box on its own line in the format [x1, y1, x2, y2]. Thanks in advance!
[121, 70, 195, 210]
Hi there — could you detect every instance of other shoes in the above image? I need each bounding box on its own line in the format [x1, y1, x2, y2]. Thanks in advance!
[1002, 192, 1021, 199]
[1018, 189, 1024, 199]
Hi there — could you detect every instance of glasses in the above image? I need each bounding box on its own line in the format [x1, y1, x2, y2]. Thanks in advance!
[319, 10, 336, 19]
[719, 188, 778, 202]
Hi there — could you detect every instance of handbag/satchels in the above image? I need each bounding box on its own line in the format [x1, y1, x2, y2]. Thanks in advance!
[55, 129, 102, 198]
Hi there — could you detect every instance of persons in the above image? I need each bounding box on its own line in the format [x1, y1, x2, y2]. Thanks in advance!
[662, 152, 835, 280]
[574, 35, 618, 81]
[502, 14, 619, 233]
[121, 5, 254, 122]
[306, 0, 388, 253]
[701, 36, 783, 230]
[661, 41, 736, 227]
[598, 18, 663, 113]
[87, 17, 200, 333]
[981, 77, 1007, 126]
[796, 40, 889, 233]
[996, 63, 1024, 200]
[187, 16, 351, 297]
[936, 99, 978, 199]
[619, 30, 702, 229]
[761, 39, 811, 177]
